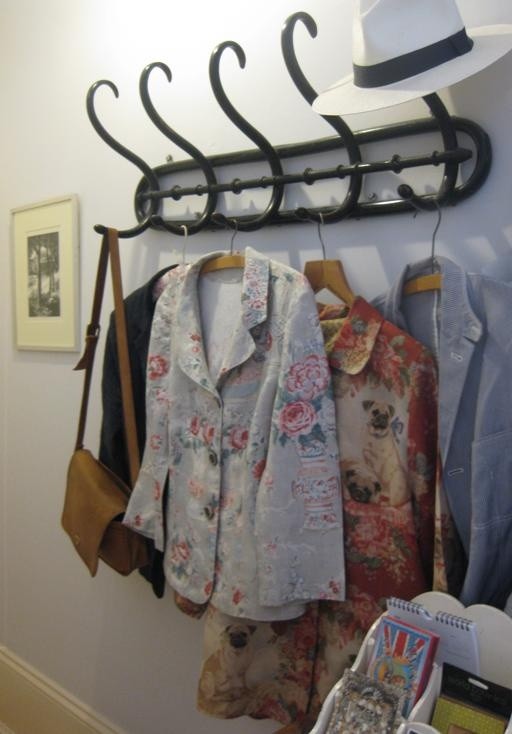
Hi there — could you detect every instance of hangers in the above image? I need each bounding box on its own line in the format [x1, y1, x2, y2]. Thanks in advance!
[401, 195, 453, 298]
[299, 211, 362, 319]
[143, 222, 200, 306]
[196, 217, 250, 276]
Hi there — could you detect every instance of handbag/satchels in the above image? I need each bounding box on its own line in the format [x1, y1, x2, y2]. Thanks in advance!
[60, 225, 152, 579]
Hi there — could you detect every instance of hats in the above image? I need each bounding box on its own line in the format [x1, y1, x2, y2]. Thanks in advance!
[311, 0, 512, 116]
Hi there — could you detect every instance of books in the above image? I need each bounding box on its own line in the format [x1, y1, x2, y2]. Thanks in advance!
[323, 666, 408, 734]
[365, 615, 441, 719]
[385, 595, 480, 677]
[430, 693, 509, 733]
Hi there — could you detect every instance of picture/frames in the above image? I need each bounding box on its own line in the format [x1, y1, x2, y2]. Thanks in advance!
[12, 194, 81, 354]
